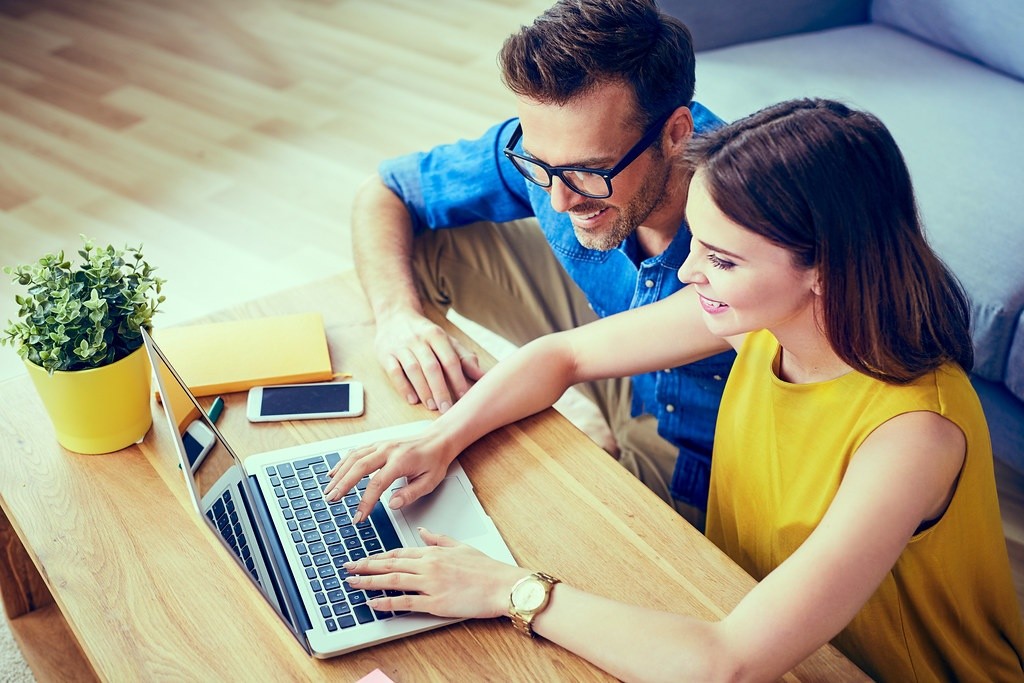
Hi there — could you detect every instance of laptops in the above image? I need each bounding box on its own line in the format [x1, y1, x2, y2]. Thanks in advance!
[136, 326, 522, 660]
[200, 465, 294, 632]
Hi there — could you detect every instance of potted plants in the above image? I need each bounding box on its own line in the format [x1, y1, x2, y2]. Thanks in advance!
[0, 233, 167, 455]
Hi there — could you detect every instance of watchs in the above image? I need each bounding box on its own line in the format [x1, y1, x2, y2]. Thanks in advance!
[510, 572, 562, 639]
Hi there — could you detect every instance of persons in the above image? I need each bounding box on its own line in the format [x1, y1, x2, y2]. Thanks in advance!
[351, 0, 737, 535]
[326, 99, 1024, 683]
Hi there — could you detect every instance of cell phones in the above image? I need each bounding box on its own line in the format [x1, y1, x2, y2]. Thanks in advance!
[247, 382, 364, 423]
[181, 419, 216, 475]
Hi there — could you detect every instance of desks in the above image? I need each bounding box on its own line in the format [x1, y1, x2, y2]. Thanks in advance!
[0, 267, 873, 683]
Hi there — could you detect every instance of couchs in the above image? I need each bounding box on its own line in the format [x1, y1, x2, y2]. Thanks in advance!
[656, 0, 1024, 480]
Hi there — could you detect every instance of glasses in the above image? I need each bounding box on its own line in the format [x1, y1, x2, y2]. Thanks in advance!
[503, 106, 679, 199]
